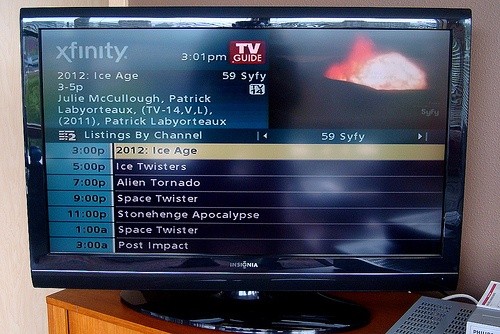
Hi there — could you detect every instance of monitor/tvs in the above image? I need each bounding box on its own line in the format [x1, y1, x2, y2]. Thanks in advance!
[20, 7, 475, 292]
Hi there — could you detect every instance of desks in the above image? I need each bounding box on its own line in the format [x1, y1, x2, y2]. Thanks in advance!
[46, 289, 424, 334]
